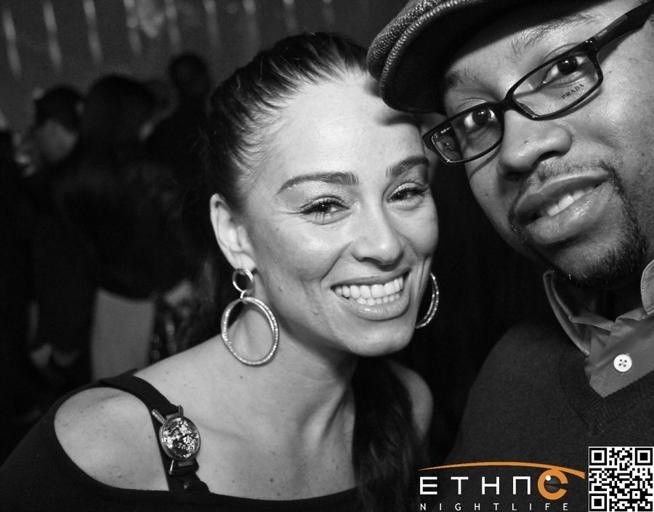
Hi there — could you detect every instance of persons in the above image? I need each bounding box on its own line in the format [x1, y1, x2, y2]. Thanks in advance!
[57, 63, 170, 378]
[1, 33, 450, 508]
[364, 0, 652, 511]
[139, 50, 238, 344]
[4, 79, 92, 408]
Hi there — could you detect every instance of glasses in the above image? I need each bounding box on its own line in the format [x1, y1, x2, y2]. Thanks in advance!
[419, 0, 654, 165]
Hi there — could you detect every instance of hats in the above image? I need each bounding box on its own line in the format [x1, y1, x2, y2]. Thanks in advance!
[363, 0, 494, 115]
